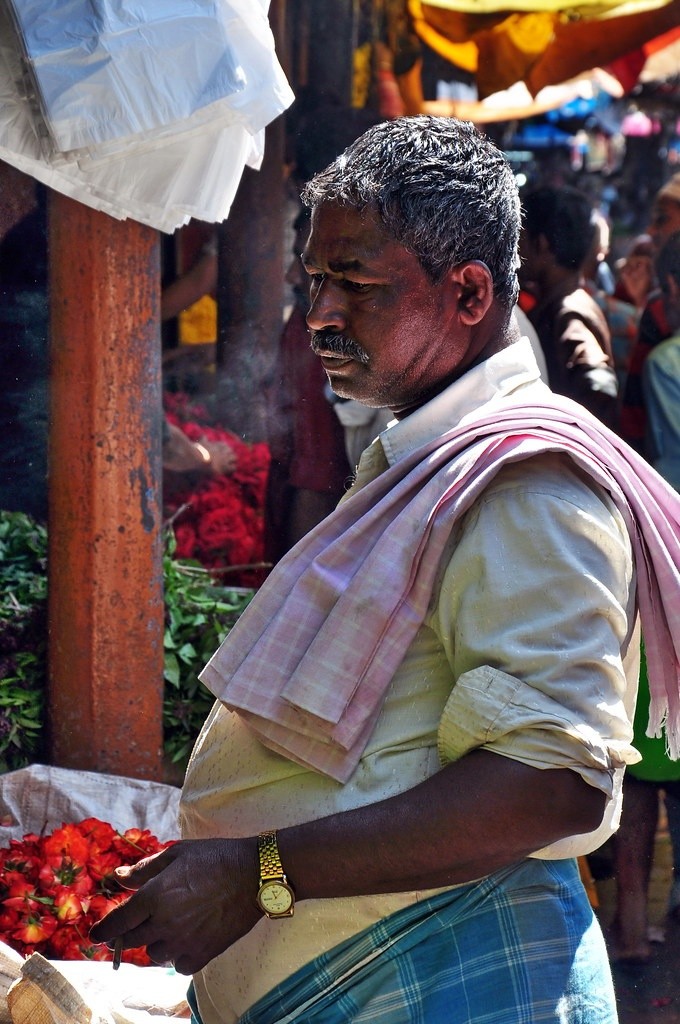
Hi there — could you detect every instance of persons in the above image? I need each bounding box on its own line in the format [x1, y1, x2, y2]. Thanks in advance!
[84, 115, 640, 1024]
[504, 103, 680, 969]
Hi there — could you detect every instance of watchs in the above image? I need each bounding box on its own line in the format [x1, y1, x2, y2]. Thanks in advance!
[257, 829, 297, 920]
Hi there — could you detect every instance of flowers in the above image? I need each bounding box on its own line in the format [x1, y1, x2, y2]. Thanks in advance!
[0, 816, 180, 969]
